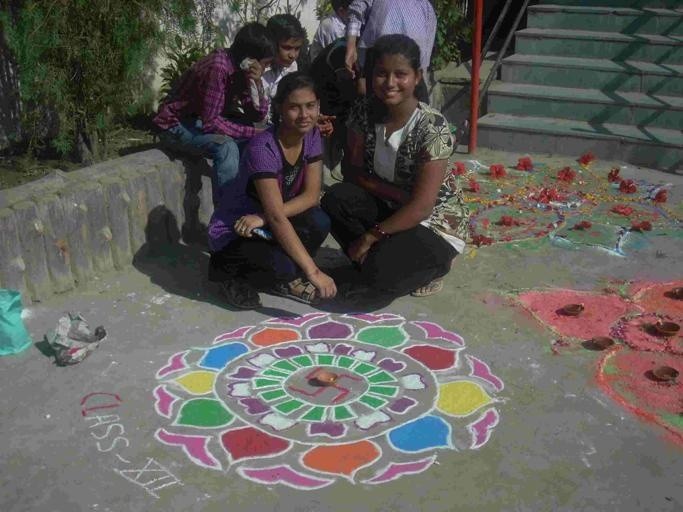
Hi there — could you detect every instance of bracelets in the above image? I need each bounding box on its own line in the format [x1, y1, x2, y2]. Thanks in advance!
[370, 224, 391, 240]
[307, 268, 320, 277]
[369, 229, 385, 242]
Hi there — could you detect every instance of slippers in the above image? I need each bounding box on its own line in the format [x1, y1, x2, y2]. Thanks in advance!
[336, 284, 368, 305]
[411, 279, 443, 296]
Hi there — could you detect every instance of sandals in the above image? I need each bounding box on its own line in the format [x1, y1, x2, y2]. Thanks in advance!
[219, 276, 263, 309]
[269, 277, 325, 305]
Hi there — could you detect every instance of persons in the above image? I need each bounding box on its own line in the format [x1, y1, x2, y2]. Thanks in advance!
[310, 0, 353, 64]
[204, 69, 338, 311]
[320, 35, 470, 298]
[152, 21, 275, 212]
[261, 13, 304, 127]
[344, 0, 439, 106]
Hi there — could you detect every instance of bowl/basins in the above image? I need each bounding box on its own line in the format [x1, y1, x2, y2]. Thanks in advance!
[673, 287, 683, 298]
[315, 372, 337, 385]
[563, 303, 583, 316]
[649, 366, 681, 381]
[656, 321, 681, 337]
[592, 335, 614, 349]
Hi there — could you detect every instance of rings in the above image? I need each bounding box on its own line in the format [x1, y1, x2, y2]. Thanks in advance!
[241, 226, 245, 232]
[234, 219, 240, 224]
